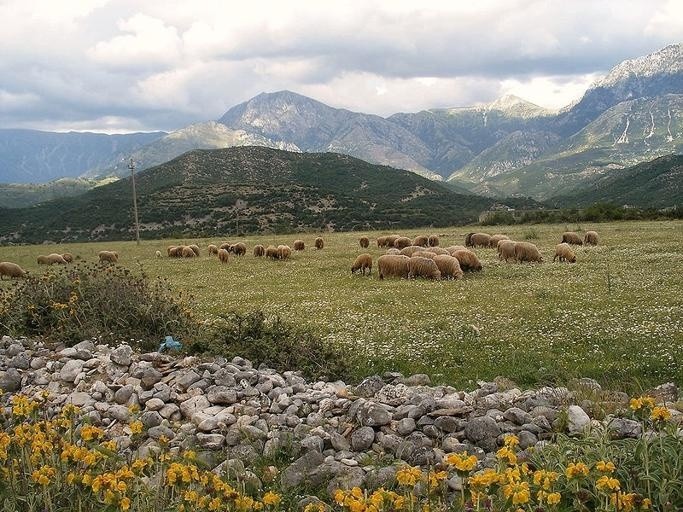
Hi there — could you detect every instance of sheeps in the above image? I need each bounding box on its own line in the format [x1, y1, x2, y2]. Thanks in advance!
[61, 253, 73, 265]
[48, 253, 67, 265]
[561, 232, 583, 246]
[167, 243, 291, 264]
[583, 231, 598, 246]
[315, 237, 324, 250]
[112, 251, 118, 259]
[294, 240, 305, 250]
[99, 251, 118, 264]
[37, 255, 50, 265]
[0, 262, 29, 281]
[351, 233, 545, 280]
[156, 251, 163, 258]
[552, 243, 576, 263]
